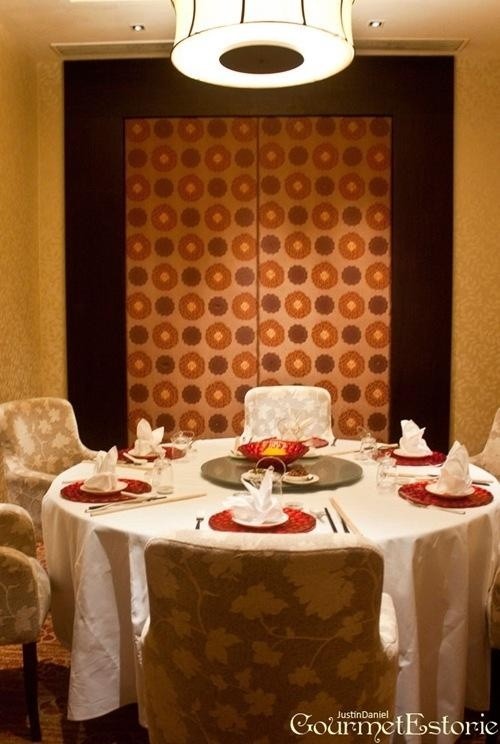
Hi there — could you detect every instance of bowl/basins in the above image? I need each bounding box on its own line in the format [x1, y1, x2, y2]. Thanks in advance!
[236, 439, 309, 469]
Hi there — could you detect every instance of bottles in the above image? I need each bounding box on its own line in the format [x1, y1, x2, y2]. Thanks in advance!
[376, 451, 398, 493]
[360, 432, 377, 464]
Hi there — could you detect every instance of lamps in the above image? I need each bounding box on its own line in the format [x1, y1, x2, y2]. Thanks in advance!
[165, 0, 358, 91]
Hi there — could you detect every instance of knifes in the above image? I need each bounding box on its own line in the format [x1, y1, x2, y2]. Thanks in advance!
[428, 474, 490, 486]
[317, 493, 367, 541]
[407, 498, 465, 516]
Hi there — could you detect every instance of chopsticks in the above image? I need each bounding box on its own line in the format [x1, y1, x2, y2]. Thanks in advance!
[90, 491, 207, 516]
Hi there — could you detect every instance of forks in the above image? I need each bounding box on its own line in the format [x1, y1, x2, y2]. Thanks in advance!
[196, 514, 205, 529]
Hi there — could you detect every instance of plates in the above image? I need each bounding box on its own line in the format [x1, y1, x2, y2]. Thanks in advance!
[231, 469, 320, 527]
[76, 425, 194, 501]
[425, 482, 476, 497]
[393, 448, 430, 457]
